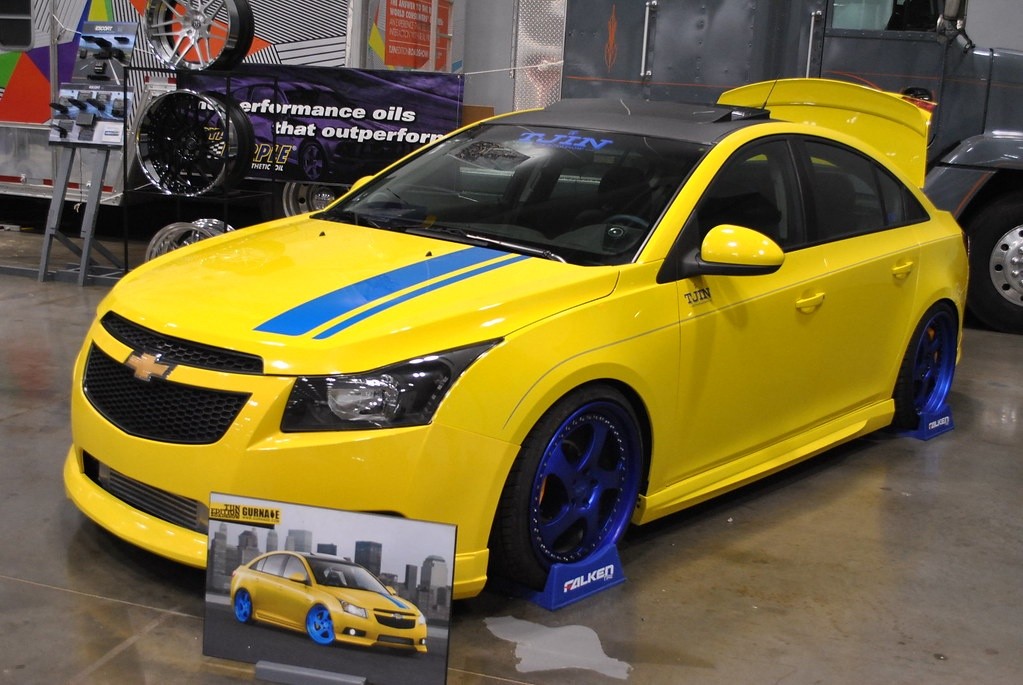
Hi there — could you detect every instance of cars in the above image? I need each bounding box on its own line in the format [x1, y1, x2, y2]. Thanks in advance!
[198, 84, 416, 179]
[231, 553, 434, 656]
[302, 136, 634, 229]
[58, 71, 975, 605]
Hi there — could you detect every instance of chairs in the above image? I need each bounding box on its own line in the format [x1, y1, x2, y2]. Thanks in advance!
[307, 566, 346, 588]
[552, 151, 904, 248]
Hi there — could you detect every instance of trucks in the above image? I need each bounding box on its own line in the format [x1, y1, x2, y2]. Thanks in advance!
[207, 1, 1022, 332]
